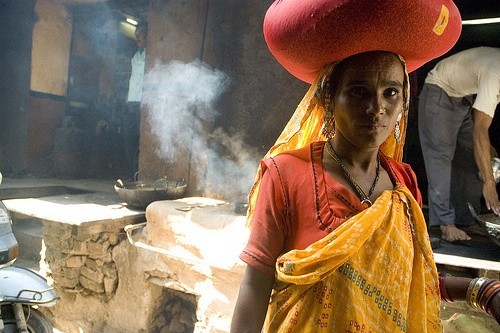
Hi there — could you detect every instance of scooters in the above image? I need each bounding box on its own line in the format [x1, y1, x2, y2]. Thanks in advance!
[0, 171, 60, 333]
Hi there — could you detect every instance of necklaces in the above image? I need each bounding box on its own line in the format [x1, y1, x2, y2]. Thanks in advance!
[324, 138, 381, 209]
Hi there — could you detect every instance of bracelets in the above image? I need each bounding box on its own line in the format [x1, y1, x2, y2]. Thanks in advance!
[438, 272, 500, 316]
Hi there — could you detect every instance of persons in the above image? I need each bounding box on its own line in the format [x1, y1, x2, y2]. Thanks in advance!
[230, 45, 500, 333]
[417, 46, 500, 241]
[125, 22, 148, 182]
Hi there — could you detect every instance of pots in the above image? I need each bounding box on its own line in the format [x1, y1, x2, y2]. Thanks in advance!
[464, 199, 499, 248]
[113, 176, 188, 210]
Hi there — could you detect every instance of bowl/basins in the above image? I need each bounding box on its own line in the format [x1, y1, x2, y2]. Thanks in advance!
[476, 155, 500, 185]
